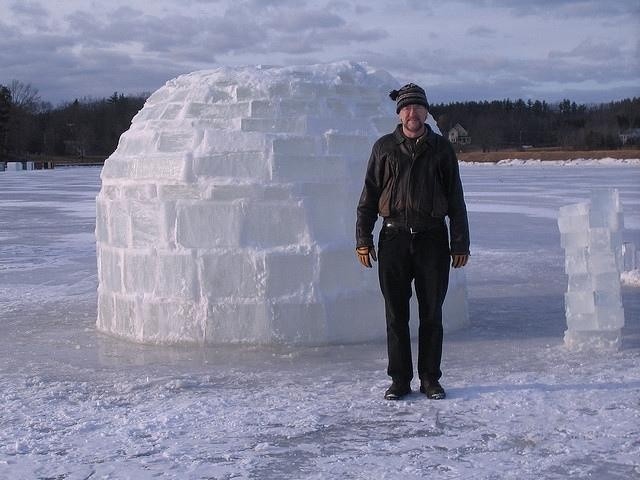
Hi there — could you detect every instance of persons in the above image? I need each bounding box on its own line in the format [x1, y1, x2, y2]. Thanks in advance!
[354, 82, 472, 401]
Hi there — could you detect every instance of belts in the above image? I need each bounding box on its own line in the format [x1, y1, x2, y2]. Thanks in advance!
[386, 224, 427, 235]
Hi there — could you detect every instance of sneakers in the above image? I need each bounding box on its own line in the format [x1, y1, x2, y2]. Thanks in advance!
[384, 379, 411, 400]
[420, 379, 445, 399]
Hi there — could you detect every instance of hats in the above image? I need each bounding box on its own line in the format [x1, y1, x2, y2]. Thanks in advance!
[389, 83, 429, 114]
